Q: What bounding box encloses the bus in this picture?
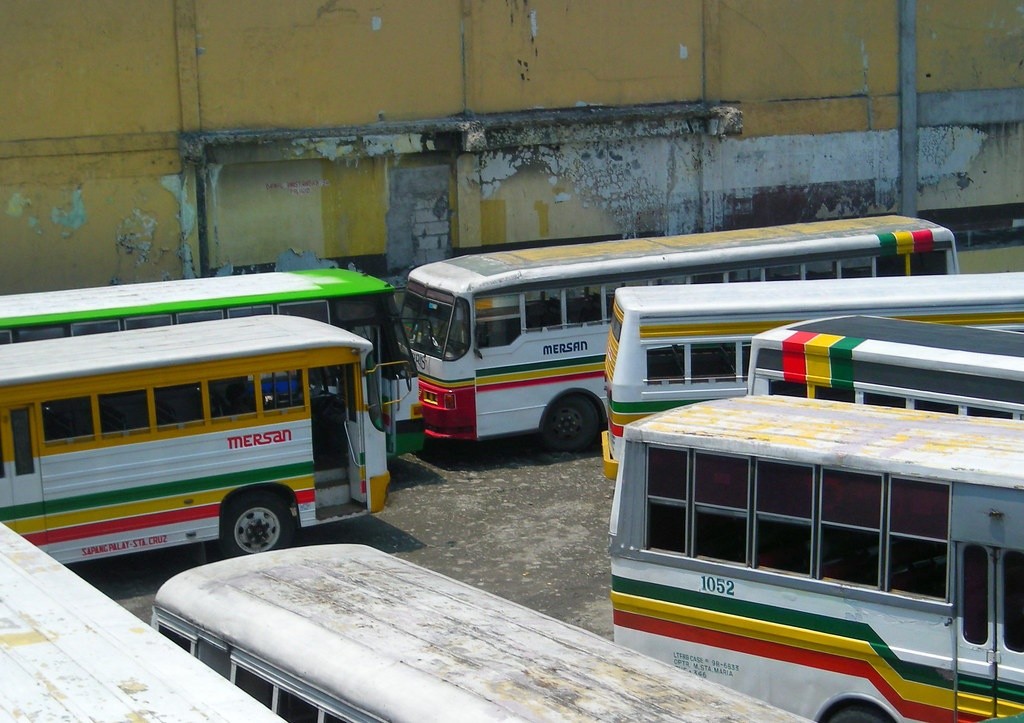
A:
[2,314,416,565]
[149,543,812,723]
[609,402,1024,723]
[1,266,439,464]
[398,216,957,456]
[2,520,287,723]
[593,272,1024,482]
[748,313,1024,417]
